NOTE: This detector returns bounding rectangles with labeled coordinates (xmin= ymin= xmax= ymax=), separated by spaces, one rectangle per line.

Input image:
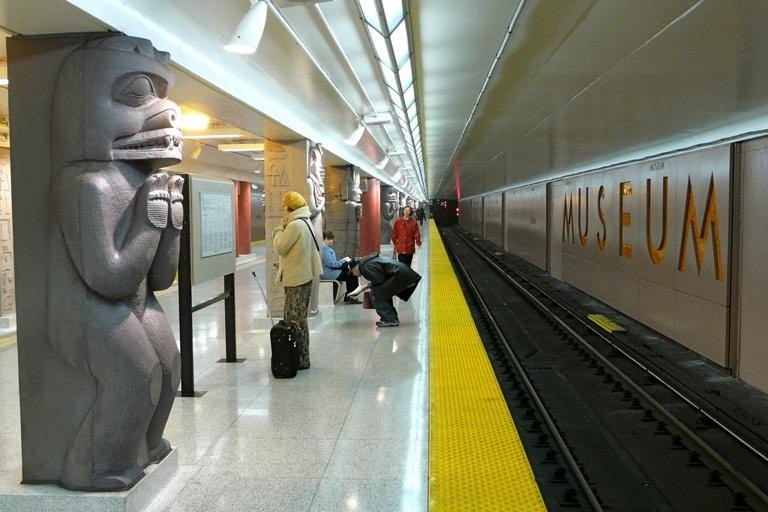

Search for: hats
xmin=282 ymin=192 xmax=306 ymax=210
xmin=349 ymin=258 xmax=360 ymax=269
xmin=324 ymin=231 xmax=335 ymax=239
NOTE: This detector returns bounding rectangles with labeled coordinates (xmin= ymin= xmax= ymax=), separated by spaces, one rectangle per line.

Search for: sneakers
xmin=375 ymin=316 xmax=401 ymax=327
xmin=344 ymin=295 xmax=362 ymax=304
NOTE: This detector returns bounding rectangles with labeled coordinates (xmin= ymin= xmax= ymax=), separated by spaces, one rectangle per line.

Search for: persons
xmin=348 ymin=252 xmax=423 ymax=328
xmin=418 ymin=207 xmax=426 ymax=225
xmin=415 ymin=208 xmax=420 ymax=223
xmin=391 ymin=206 xmax=422 ymax=268
xmin=319 ymin=230 xmax=364 ymax=305
xmin=272 ymin=191 xmax=324 ymax=370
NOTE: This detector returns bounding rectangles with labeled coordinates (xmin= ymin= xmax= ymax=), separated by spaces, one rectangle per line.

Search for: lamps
xmin=224 ymin=1 xmax=268 ymax=55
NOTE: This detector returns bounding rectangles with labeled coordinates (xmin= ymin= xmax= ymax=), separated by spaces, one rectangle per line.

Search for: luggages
xmin=269 ymin=320 xmax=299 ymax=379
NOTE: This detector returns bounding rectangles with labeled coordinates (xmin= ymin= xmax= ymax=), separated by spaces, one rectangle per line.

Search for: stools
xmin=320 ymin=275 xmax=345 ymax=310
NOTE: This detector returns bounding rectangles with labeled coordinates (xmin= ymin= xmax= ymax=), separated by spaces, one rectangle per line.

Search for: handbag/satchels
xmin=363 ymin=291 xmax=375 ymax=310
